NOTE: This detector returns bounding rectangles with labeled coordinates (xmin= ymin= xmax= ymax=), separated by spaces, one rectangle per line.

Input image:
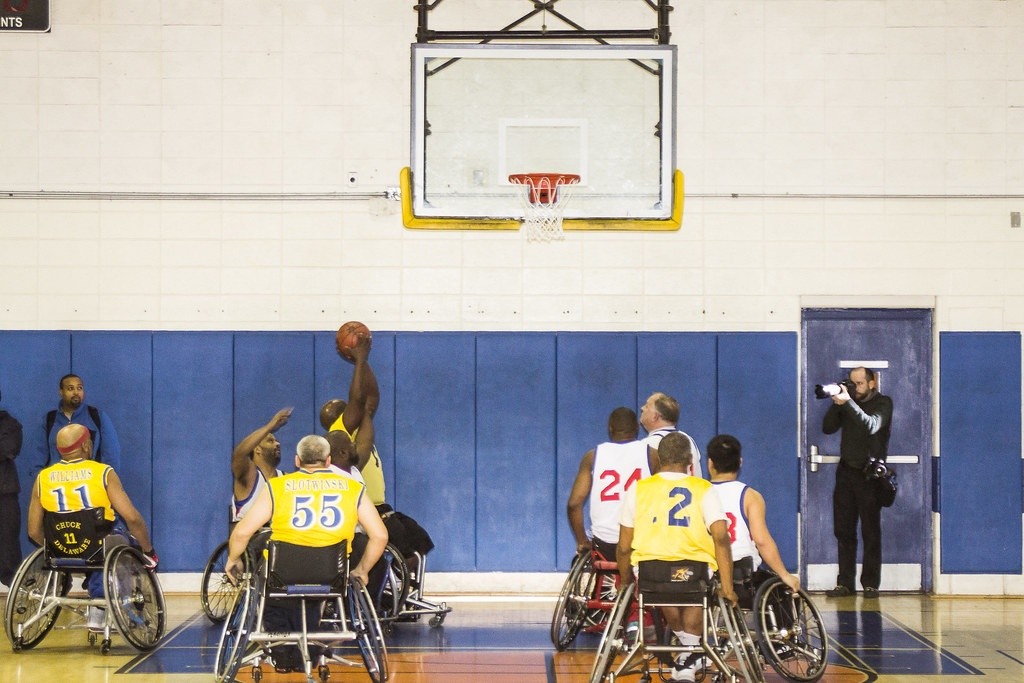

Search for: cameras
xmin=815 ymin=380 xmax=857 ymax=400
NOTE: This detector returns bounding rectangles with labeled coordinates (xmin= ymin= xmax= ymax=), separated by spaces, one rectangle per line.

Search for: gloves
xmin=143 ymin=549 xmax=158 ymax=572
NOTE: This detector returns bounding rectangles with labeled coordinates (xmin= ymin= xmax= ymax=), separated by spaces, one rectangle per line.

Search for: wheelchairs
xmin=202 ymin=505 xmax=453 ymax=639
xmin=588 ymin=559 xmax=766 ymax=683
xmin=550 ymin=538 xmax=826 ymax=683
xmin=214 ymin=538 xmax=390 ymax=683
xmin=4 ymin=506 xmax=167 ymax=655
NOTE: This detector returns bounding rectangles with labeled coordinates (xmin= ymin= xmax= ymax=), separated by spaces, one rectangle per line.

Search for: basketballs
xmin=336 ymin=321 xmax=373 ymax=359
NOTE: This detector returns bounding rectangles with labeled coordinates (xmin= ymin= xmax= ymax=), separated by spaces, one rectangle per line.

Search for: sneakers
xmin=825 ymin=585 xmax=852 ymax=597
xmin=86 ymin=606 xmax=123 ymax=629
xmin=627 ymin=621 xmax=639 ymax=643
xmin=864 ymin=587 xmax=879 ymax=599
xmin=0 ymin=581 xmax=23 ymax=596
xmin=671 ymin=660 xmax=695 ymax=683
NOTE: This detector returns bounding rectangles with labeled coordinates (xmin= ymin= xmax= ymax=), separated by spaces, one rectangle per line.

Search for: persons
xmin=701 ymin=435 xmax=807 ymax=662
xmin=615 ymin=433 xmax=732 ymax=683
xmin=30 ymin=423 xmax=158 ymax=633
xmin=30 ymin=374 xmax=120 ymax=588
xmin=566 ymin=392 xmax=702 ymax=641
xmin=0 ymin=391 xmax=23 ymax=593
xmin=232 ymin=337 xmax=435 ymax=673
xmin=822 ymin=367 xmax=893 ymax=598
